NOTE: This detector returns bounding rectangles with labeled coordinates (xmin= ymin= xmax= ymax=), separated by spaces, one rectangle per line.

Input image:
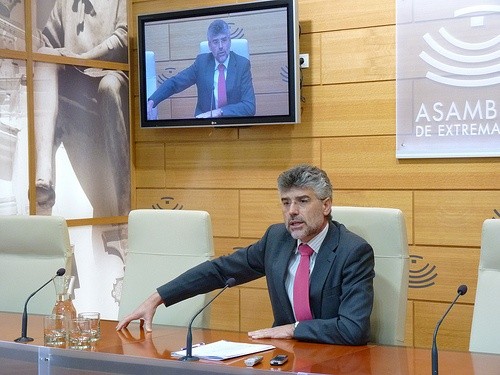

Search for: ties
xmin=217 ymin=63 xmax=227 ymax=108
xmin=293 ymin=243 xmax=315 ymax=321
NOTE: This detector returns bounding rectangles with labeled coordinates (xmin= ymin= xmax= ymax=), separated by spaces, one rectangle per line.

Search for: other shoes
xmin=28 ymin=182 xmax=55 ymax=210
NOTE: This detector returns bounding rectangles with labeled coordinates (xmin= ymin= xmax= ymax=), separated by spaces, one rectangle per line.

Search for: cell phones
xmin=270 ymin=355 xmax=288 ymax=366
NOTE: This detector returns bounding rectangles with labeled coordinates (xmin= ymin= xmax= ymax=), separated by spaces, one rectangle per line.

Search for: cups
xmin=43 ymin=315 xmax=66 ymax=348
xmin=79 ymin=312 xmax=100 ymax=342
xmin=68 ymin=318 xmax=91 ymax=349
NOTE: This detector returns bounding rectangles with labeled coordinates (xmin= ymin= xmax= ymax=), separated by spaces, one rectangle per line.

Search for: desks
xmin=0 ymin=312 xmax=500 ymax=375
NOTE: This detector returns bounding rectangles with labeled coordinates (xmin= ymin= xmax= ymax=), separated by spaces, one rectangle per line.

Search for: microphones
xmin=210 ymin=86 xmax=215 ymax=117
xmin=181 ymin=278 xmax=237 ymax=361
xmin=15 ymin=268 xmax=66 ymax=342
xmin=431 ymin=284 xmax=468 ymax=375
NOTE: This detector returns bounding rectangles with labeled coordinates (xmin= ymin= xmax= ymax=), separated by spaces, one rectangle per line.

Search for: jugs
xmin=51 ymin=274 xmax=76 ymax=338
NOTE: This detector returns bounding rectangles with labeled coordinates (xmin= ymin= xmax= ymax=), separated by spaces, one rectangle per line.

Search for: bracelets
xmin=220 ymin=108 xmax=223 ymax=116
xmin=295 ymin=321 xmax=299 ymax=328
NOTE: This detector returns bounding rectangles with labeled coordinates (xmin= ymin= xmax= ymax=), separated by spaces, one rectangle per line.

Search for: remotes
xmin=244 ymin=356 xmax=263 ymax=366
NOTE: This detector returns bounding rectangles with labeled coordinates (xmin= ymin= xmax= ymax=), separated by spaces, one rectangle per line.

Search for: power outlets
xmin=299 ymin=54 xmax=309 ymax=67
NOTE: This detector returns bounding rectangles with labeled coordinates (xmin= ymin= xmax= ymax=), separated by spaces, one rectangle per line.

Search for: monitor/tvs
xmin=138 ymin=0 xmax=301 ymax=128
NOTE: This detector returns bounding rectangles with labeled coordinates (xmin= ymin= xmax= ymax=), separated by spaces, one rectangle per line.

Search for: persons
xmin=43 ymin=0 xmax=130 ymax=215
xmin=115 ymin=165 xmax=375 ymax=345
xmin=147 ymin=20 xmax=256 ymax=117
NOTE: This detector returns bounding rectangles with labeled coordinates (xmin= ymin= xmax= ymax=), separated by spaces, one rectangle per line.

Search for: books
xmin=171 ymin=340 xmax=276 ymax=361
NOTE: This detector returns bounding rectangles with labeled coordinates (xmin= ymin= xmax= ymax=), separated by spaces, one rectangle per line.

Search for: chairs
xmin=330 ymin=207 xmax=410 ymax=345
xmin=469 ymin=219 xmax=500 ymax=354
xmin=0 ymin=215 xmax=72 ymax=314
xmin=118 ymin=209 xmax=214 ymax=329
